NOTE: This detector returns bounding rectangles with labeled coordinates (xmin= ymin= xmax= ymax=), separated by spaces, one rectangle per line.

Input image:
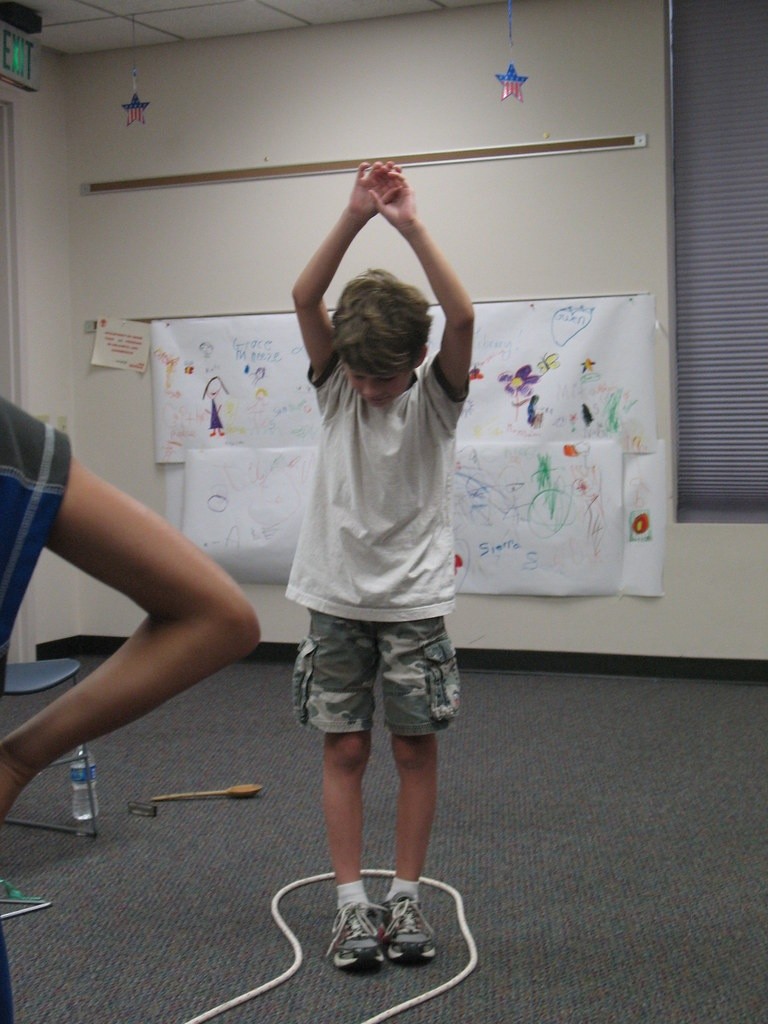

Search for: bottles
xmin=70 ymin=744 xmax=99 ymax=821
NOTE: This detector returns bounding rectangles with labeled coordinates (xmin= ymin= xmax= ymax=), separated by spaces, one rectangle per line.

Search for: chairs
xmin=1 ymin=659 xmax=97 ymax=835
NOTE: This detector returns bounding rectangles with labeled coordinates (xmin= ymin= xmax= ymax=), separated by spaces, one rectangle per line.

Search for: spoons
xmin=151 ymin=784 xmax=263 ymax=802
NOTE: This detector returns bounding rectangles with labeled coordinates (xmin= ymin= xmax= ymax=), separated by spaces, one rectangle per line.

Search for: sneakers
xmin=380 ymin=892 xmax=437 ymax=961
xmin=326 ymin=901 xmax=384 ymax=969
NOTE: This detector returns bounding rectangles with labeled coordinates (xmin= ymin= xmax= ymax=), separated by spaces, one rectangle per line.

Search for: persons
xmin=292 ymin=160 xmax=474 ymax=974
xmin=1 ymin=396 xmax=261 ymax=1024
